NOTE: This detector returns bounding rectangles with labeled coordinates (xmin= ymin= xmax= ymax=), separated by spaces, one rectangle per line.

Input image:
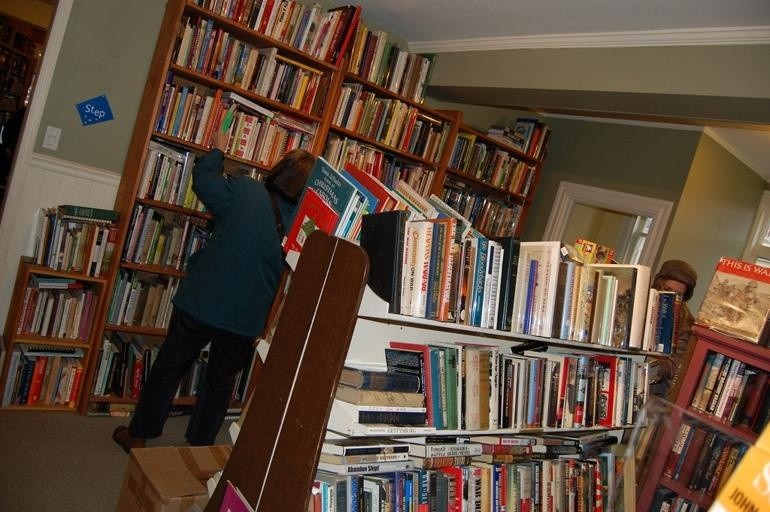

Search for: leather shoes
xmin=113 ymin=425 xmax=145 ymax=453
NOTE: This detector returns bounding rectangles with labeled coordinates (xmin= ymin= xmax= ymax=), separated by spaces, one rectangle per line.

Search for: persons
xmin=112 ymin=129 xmax=315 ymax=459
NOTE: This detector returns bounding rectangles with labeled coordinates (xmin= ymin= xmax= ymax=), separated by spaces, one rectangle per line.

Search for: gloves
xmin=650 ymin=357 xmax=676 ymax=381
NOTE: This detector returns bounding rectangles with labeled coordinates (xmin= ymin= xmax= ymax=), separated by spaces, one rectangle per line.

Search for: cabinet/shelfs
xmin=0 ymin=0 xmax=770 ymax=512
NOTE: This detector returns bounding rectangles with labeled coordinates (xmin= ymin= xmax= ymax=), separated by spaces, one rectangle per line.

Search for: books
xmin=325 ymin=22 xmax=550 ymax=239
xmin=0 ymin=204 xmax=120 ymax=410
xmin=88 ymin=140 xmax=264 ymax=402
xmin=241 ymin=156 xmax=769 ymax=512
xmin=156 ymin=1 xmax=362 ymax=169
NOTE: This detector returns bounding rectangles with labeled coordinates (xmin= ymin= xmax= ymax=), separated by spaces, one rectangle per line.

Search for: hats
xmin=655 ymin=260 xmax=698 ymax=289
